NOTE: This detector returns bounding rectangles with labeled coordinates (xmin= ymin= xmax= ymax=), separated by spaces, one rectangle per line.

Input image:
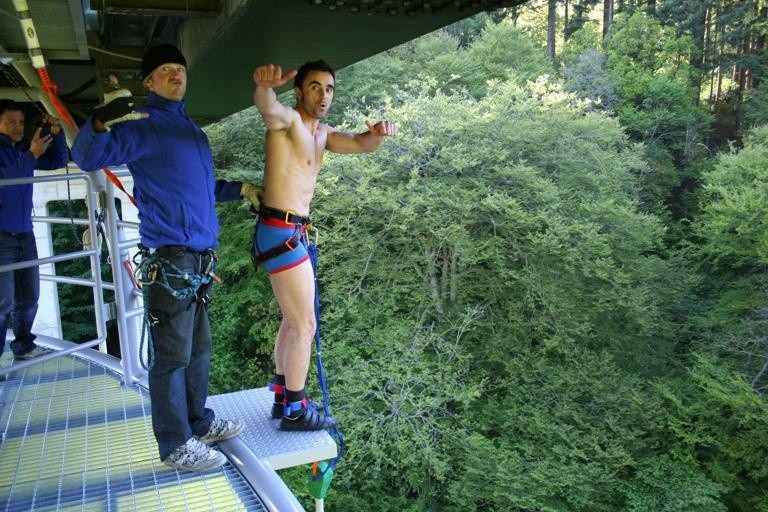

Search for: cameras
xmin=37 ymin=119 xmax=52 ymax=138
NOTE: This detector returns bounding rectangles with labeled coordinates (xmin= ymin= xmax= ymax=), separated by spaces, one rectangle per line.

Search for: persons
xmin=247 ymin=60 xmax=404 ymax=434
xmin=0 ymin=99 xmax=69 ymax=367
xmin=66 ymin=44 xmax=264 ymax=474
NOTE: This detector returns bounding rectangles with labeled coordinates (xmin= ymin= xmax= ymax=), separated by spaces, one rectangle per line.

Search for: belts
xmin=0 ymin=230 xmax=33 ymax=240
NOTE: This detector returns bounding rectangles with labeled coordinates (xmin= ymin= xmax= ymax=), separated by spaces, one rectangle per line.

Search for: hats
xmin=140 ymin=43 xmax=188 ymax=88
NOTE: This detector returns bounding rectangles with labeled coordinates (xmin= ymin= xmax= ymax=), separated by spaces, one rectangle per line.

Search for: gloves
xmin=92 ymin=81 xmax=144 ymax=133
xmin=239 ymin=183 xmax=264 ymax=210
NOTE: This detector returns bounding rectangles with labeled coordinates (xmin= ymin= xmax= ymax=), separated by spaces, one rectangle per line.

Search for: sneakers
xmin=281 ymin=403 xmax=337 ymax=431
xmin=161 ymin=437 xmax=228 ymax=473
xmin=193 ymin=417 xmax=245 ymax=445
xmin=14 ymin=346 xmax=53 ymax=361
xmin=269 ymin=381 xmax=285 ymax=419
